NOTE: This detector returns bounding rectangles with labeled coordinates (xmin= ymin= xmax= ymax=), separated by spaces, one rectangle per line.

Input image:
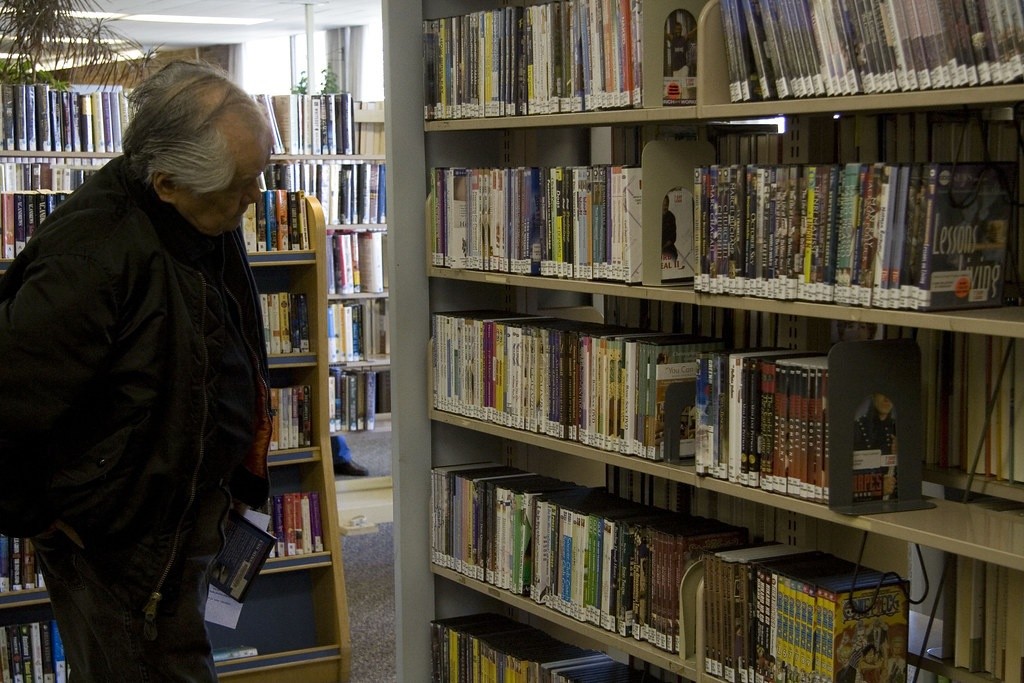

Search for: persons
xmin=667 ymin=23 xmax=697 ymax=78
xmin=0 ymin=59 xmax=274 ymax=683
xmin=853 ymin=392 xmax=897 ymax=500
xmin=662 ymin=195 xmax=678 ymax=262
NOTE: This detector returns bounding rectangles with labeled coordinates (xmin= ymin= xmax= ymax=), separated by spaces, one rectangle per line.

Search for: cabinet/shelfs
xmin=0 ymin=0 xmax=1024 ymax=683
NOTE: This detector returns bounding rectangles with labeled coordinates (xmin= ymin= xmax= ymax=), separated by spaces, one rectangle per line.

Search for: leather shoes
xmin=335 ymin=460 xmax=369 ymax=476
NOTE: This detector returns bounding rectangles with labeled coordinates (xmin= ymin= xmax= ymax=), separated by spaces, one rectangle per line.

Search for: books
xmin=0 ymin=619 xmax=71 ymax=683
xmin=0 ymin=157 xmax=390 ymax=453
xmin=0 ymin=532 xmax=46 ymax=591
xmin=421 ymin=0 xmax=1024 ymax=683
xmin=208 ymin=492 xmax=326 ymax=603
xmin=0 ymin=82 xmax=386 ymax=156
xmin=213 ymin=644 xmax=258 ymax=663
